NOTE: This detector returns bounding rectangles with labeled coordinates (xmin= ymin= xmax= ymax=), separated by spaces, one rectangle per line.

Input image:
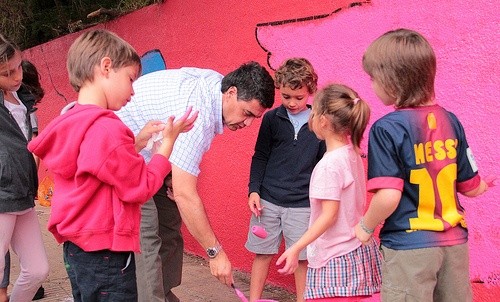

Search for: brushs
xmin=483 ymin=173 xmax=500 ymax=188
xmin=251 ymin=208 xmax=267 ymax=239
xmin=231 ymin=282 xmax=249 ymax=302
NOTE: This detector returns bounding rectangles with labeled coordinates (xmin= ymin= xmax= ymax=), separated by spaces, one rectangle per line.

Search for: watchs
xmin=206 ymin=244 xmax=221 ymax=258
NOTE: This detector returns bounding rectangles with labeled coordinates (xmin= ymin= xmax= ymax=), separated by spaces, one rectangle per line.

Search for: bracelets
xmin=360 ymin=218 xmax=374 ymax=233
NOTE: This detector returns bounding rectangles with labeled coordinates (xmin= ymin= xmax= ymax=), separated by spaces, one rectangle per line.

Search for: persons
xmin=276 ymin=84 xmax=384 ymax=302
xmin=111 ymin=60 xmax=274 ymax=302
xmin=0 ymin=34 xmax=49 ymax=302
xmin=244 ymin=56 xmax=327 ymax=302
xmin=26 ymin=29 xmax=199 ymax=302
xmin=353 ymin=28 xmax=488 ymax=302
